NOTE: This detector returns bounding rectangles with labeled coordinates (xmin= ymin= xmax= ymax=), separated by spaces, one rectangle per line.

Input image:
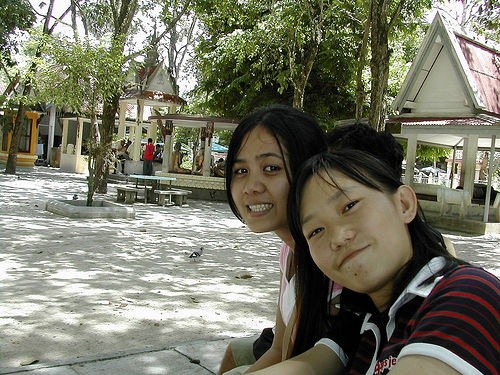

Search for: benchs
xmin=124 ymin=160 xmax=162 ymax=175
xmin=114 ymin=185 xmax=192 ymax=207
xmin=156 ymin=171 xmax=227 ymax=202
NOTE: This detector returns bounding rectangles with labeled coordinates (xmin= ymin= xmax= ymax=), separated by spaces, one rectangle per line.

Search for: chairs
xmin=436 ymin=188 xmax=480 ymax=219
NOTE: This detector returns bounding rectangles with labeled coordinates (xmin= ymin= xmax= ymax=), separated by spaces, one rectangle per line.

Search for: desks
xmin=128 ymin=175 xmax=176 ymax=202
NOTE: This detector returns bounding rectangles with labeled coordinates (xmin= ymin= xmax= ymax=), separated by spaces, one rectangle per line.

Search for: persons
xmin=140 ymin=143 xmax=144 ymax=161
xmin=116 ymin=139 xmax=134 ymax=160
xmin=143 ymin=139 xmax=154 ymax=176
xmin=224 ymin=105 xmax=344 ymax=375
xmin=154 ymin=144 xmax=161 ymax=160
xmin=248 ymin=149 xmax=500 ymax=375
xmin=170 ymin=142 xmax=191 ymax=174
xmin=192 ymin=147 xmax=204 ymax=175
xmin=211 ymin=158 xmax=224 ymax=177
xmin=218 ymin=124 xmax=403 ymax=375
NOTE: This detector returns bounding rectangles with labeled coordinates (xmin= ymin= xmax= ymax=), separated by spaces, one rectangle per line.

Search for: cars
xmin=419 ymin=165 xmax=447 ymax=177
xmin=402 ymin=159 xmax=420 ymax=176
